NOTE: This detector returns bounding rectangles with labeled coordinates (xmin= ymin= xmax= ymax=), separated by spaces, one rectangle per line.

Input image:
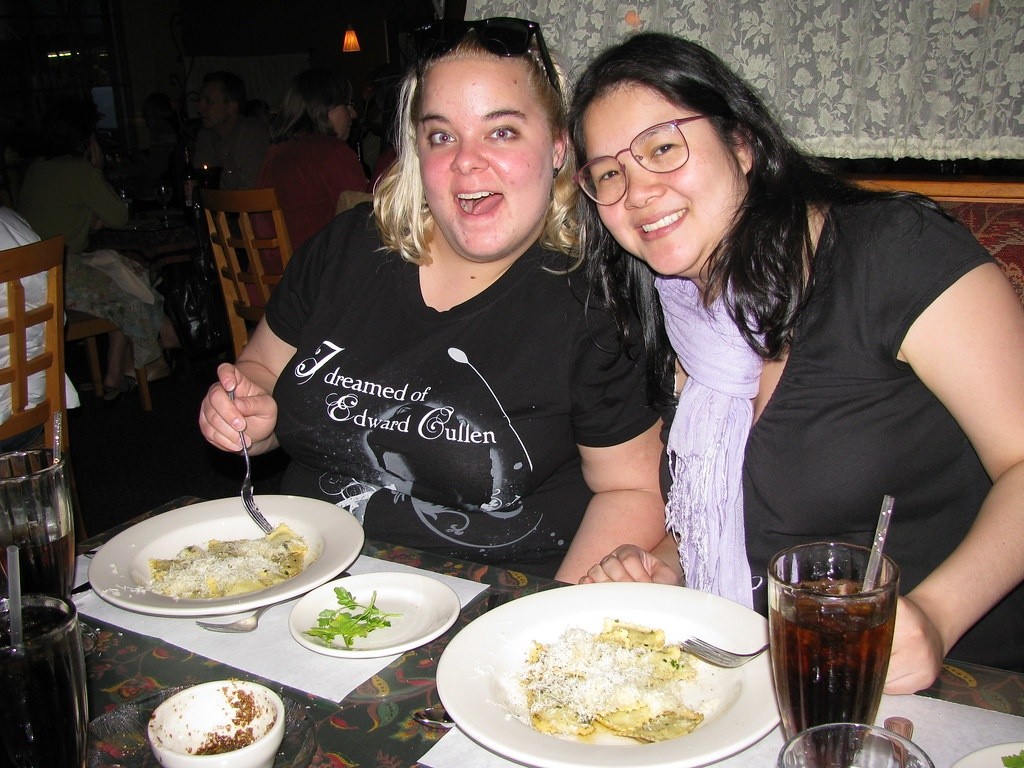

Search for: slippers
xmin=104 ymin=382 xmax=129 ymax=400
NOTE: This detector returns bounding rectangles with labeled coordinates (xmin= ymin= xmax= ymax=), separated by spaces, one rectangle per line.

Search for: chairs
xmin=0 ymin=236 xmax=87 ymax=543
xmin=193 ymin=187 xmax=292 ymax=358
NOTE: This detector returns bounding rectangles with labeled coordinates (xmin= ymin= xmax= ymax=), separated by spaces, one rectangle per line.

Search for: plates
xmin=951 ymin=742 xmax=1024 ymax=768
xmin=88 ymin=497 xmax=365 ymax=615
xmin=288 ymin=573 xmax=461 ymax=659
xmin=436 ymin=583 xmax=782 ymax=768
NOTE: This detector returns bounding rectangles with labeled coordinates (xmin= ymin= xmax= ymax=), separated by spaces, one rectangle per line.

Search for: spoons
xmin=412 ymin=708 xmax=455 ymax=729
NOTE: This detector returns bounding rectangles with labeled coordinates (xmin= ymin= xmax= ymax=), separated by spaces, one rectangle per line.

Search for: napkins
xmin=418 ymin=685 xmax=1024 ymax=768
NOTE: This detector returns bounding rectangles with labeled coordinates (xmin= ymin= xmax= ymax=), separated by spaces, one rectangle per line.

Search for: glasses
xmin=572 ymin=112 xmax=726 ymax=207
xmin=409 ymin=17 xmax=562 ymax=96
xmin=337 ymin=101 xmax=355 ymax=113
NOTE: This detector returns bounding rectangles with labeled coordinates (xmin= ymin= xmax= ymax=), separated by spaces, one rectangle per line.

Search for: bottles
xmin=179 ymin=145 xmax=200 ymax=206
xmin=355 ymin=141 xmax=369 ymax=178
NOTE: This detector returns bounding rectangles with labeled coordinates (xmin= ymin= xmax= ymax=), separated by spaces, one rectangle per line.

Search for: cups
xmin=117 ymin=188 xmax=134 ymax=208
xmin=0 ymin=448 xmax=76 ymax=604
xmin=767 ymin=541 xmax=900 ymax=768
xmin=0 ymin=593 xmax=90 ymax=767
xmin=777 ymin=722 xmax=935 ymax=768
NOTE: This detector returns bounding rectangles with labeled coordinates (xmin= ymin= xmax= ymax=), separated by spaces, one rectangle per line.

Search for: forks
xmin=229 ymin=391 xmax=274 ymax=535
xmin=195 ymin=572 xmax=351 ymax=634
xmin=681 ymin=636 xmax=769 ymax=668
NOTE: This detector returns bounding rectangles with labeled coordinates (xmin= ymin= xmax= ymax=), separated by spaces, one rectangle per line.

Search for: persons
xmin=0 ymin=67 xmax=370 ymax=453
xmin=566 ymin=33 xmax=1024 ymax=695
xmin=199 ymin=17 xmax=666 ymax=585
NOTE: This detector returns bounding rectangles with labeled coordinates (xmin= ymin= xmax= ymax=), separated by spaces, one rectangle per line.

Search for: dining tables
xmin=65 ymin=492 xmax=1024 ymax=768
xmin=69 ymin=543 xmax=491 ymax=705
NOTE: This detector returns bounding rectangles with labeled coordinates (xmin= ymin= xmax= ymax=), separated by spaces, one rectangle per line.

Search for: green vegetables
xmin=304 ymin=586 xmax=404 ymax=649
xmin=1001 ymin=750 xmax=1024 ymax=768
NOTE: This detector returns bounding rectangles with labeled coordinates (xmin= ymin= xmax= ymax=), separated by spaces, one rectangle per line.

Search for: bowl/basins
xmin=148 ymin=679 xmax=285 ymax=767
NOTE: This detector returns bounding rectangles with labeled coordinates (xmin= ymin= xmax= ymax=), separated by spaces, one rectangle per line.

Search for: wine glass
xmin=153 ymin=180 xmax=173 ymax=225
